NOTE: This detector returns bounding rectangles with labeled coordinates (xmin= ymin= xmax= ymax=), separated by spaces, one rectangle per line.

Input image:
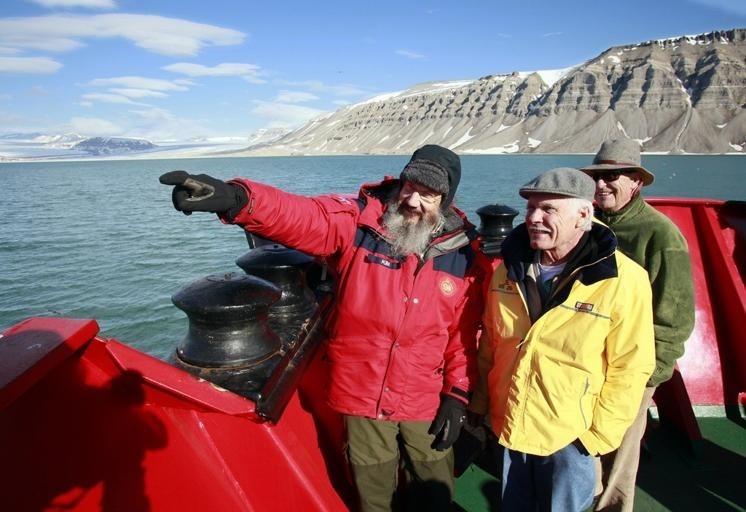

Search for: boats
xmin=0 ymin=197 xmax=746 ymax=510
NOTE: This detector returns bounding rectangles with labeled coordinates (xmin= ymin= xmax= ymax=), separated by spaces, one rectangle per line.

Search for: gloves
xmin=158 ymin=170 xmax=239 ymax=216
xmin=428 ymin=395 xmax=467 ymax=451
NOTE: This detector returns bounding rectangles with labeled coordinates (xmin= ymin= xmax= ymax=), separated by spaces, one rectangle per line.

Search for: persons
xmin=575 ymin=136 xmax=695 ymax=512
xmin=158 ymin=142 xmax=494 ymax=512
xmin=476 ymin=165 xmax=658 ymax=512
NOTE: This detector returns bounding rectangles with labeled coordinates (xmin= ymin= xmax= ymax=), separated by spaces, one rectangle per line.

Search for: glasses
xmin=590 ymin=168 xmax=638 ymax=182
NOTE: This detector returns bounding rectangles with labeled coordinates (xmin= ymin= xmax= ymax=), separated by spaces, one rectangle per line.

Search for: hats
xmin=519 ymin=167 xmax=597 ymax=200
xmin=399 ymin=144 xmax=462 ymax=212
xmin=579 ymin=139 xmax=655 ymax=187
